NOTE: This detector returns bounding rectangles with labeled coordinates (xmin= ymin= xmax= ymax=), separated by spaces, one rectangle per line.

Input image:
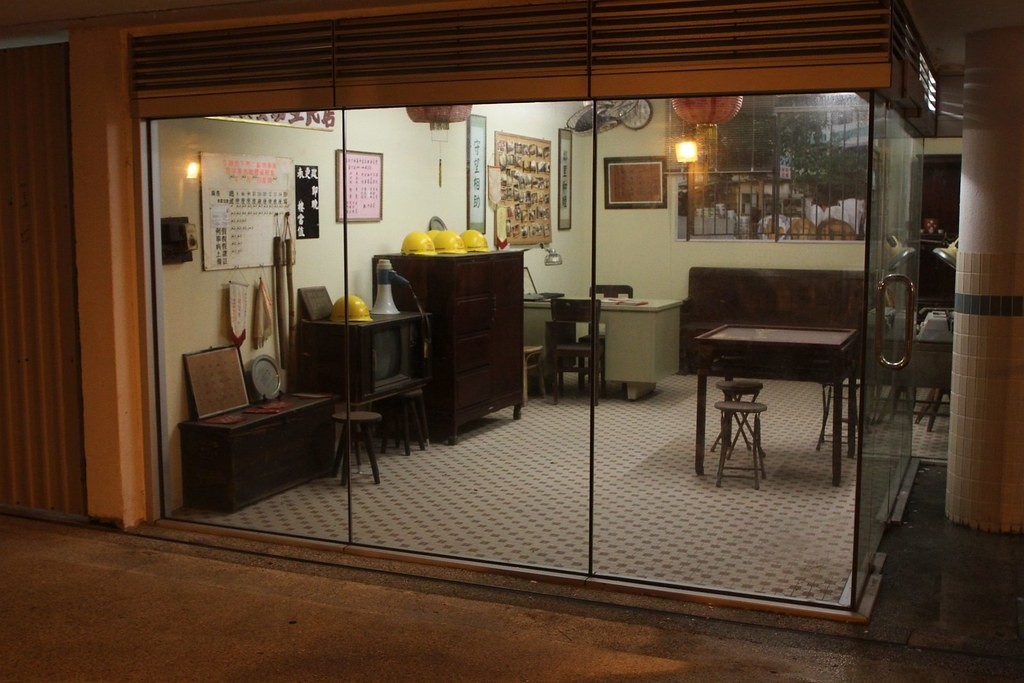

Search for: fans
xmin=566 ymin=99 xmax=640 ymax=136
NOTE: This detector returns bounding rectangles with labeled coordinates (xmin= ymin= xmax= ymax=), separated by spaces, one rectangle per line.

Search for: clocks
xmin=619 ymin=99 xmax=653 ymax=130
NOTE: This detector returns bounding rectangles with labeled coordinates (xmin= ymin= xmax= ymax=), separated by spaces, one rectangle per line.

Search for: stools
xmin=711 ymin=381 xmax=766 ymax=457
xmin=381 ymin=389 xmax=425 ymax=456
xmin=816 ymin=378 xmax=864 ymax=458
xmin=331 ymin=411 xmax=382 ymax=485
xmin=714 ymin=401 xmax=767 ymax=490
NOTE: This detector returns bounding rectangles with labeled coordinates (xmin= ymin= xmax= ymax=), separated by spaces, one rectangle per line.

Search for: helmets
xmin=401 ymin=229 xmax=490 ymax=255
xmin=329 ymin=295 xmax=373 ymax=323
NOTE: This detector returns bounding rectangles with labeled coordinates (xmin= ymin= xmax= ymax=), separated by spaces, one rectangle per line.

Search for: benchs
xmin=680 ymin=266 xmax=865 ymax=379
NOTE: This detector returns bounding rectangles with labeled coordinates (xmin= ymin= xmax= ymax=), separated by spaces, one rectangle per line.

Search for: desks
xmin=523 ymin=296 xmax=682 ymax=400
xmin=694 ymin=324 xmax=859 ymax=485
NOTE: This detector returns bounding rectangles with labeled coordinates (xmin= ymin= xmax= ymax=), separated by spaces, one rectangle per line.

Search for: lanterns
xmin=670 ymin=96 xmax=743 ymax=169
xmin=405 ymin=105 xmax=471 ymax=189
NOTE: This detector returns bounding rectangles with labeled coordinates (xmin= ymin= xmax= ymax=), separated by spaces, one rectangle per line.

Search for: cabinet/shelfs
xmin=371 ymin=250 xmax=523 ymax=445
xmin=177 ymin=392 xmax=336 ymax=512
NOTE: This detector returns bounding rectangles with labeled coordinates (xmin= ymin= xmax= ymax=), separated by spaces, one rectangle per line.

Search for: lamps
xmin=524 ymin=242 xmax=562 ymax=266
xmin=932 ymin=238 xmax=958 ymax=269
xmin=881 ymin=236 xmax=916 ymax=307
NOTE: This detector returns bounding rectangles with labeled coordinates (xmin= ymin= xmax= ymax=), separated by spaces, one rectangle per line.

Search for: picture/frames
xmin=604 ymin=155 xmax=668 ymax=209
xmin=183 ymin=345 xmax=249 ymax=421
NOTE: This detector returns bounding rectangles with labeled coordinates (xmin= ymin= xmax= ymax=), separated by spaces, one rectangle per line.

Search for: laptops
xmin=524 ymin=267 xmax=565 ymax=299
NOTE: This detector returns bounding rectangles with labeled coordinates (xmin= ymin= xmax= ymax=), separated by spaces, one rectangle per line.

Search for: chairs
xmin=578 ymin=285 xmax=634 ymax=393
xmin=550 ymin=298 xmax=605 ymax=407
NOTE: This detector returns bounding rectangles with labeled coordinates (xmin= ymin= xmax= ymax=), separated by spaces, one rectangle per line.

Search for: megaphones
xmin=368 ymin=260 xmax=410 ymax=314
